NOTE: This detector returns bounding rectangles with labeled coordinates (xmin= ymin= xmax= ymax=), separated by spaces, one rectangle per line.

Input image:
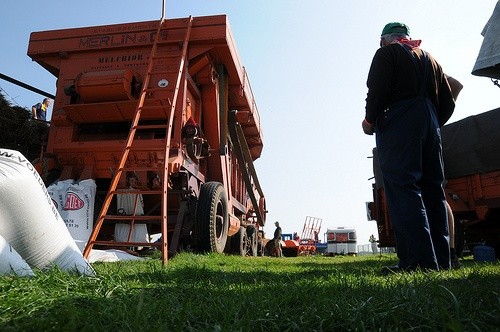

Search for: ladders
xmin=82 ymin=15 xmax=193 ymax=269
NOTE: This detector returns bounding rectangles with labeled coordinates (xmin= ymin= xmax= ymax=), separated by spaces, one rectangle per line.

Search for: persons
xmin=369 ymin=234 xmax=377 ymax=254
xmin=31 ymin=97 xmax=51 ymax=121
xmin=274 ymin=221 xmax=283 ymax=257
xmin=361 ymin=22 xmax=456 ymax=273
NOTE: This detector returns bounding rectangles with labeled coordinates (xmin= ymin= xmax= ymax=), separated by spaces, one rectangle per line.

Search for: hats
xmin=380 ymin=22 xmax=410 ymax=46
